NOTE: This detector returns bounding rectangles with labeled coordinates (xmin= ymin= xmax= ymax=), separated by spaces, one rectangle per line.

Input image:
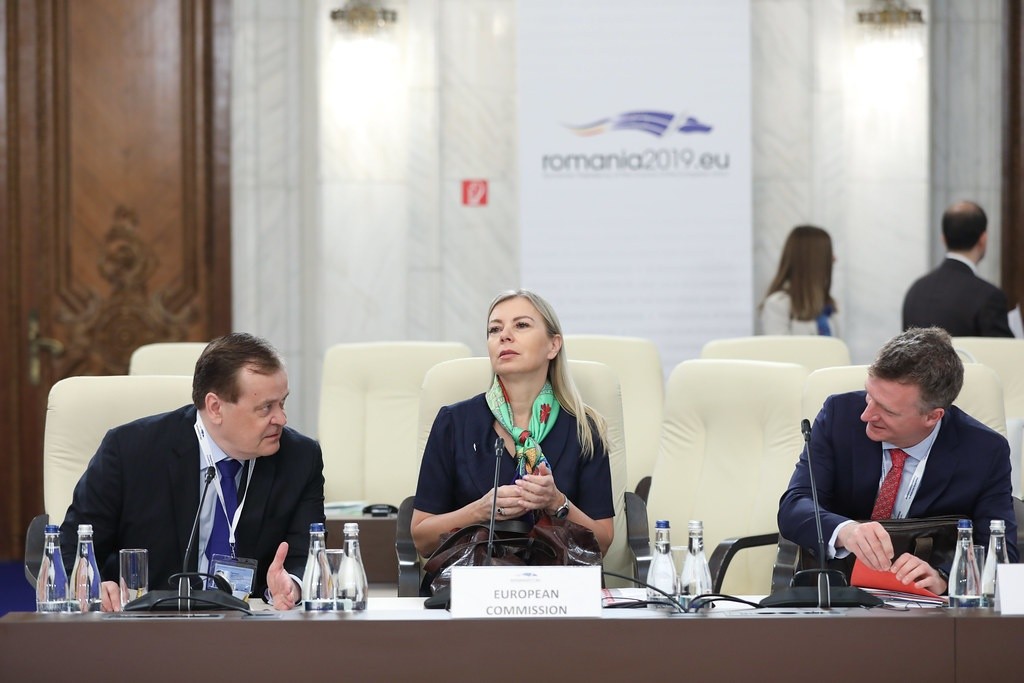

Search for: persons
xmin=776 ymin=325 xmax=1019 ymax=596
xmin=901 ymin=199 xmax=1016 ymax=337
xmin=760 ymin=225 xmax=838 ymax=338
xmin=409 ymin=288 xmax=616 ymax=597
xmin=48 ymin=331 xmax=327 ymax=612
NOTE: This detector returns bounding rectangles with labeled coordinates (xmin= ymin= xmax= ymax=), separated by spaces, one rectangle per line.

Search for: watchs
xmin=545 ymin=493 xmax=570 ymax=521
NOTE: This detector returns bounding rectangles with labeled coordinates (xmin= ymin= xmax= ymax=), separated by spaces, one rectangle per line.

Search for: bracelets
xmin=937 ymin=567 xmax=949 ymax=586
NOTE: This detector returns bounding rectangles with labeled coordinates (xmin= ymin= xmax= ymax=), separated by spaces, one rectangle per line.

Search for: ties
xmin=870 ymin=448 xmax=910 ymax=520
xmin=204 ymin=459 xmax=242 ymax=590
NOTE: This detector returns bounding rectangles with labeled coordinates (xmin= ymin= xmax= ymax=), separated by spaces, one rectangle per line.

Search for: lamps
xmin=330 ymin=1 xmax=396 ymax=39
xmin=857 ymin=0 xmax=925 ymax=34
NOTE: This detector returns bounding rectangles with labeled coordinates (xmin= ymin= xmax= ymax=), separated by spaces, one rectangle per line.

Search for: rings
xmin=496 ymin=507 xmax=506 ymax=516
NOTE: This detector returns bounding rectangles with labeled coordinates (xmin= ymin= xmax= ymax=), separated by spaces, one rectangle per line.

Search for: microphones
xmin=121 ymin=465 xmax=251 ymax=611
xmin=424 ymin=437 xmax=506 ymax=611
xmin=760 ymin=419 xmax=884 ymax=607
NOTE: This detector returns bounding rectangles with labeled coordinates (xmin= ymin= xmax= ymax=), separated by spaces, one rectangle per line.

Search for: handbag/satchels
xmin=801 ymin=515 xmax=974 ymax=586
xmin=424 ymin=517 xmax=605 ymax=609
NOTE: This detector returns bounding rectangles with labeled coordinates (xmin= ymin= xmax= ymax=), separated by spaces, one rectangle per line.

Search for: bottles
xmin=645 ymin=521 xmax=678 ymax=610
xmin=302 ymin=522 xmax=333 ymax=610
xmin=333 ymin=523 xmax=368 ymax=610
xmin=679 ymin=520 xmax=713 ymax=611
xmin=979 ymin=519 xmax=1009 ymax=607
xmin=35 ymin=525 xmax=68 ymax=613
xmin=948 ymin=519 xmax=980 ymax=607
xmin=69 ymin=525 xmax=102 ymax=614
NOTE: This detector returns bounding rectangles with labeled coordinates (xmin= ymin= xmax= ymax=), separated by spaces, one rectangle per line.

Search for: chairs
xmin=315 ymin=333 xmax=1024 ymax=598
xmin=42 ymin=336 xmax=209 ymax=529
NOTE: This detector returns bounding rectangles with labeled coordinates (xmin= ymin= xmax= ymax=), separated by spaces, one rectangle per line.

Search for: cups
xmin=974 ymin=545 xmax=984 ymax=572
xmin=324 ymin=547 xmax=344 ymax=583
xmin=120 ymin=548 xmax=149 ymax=611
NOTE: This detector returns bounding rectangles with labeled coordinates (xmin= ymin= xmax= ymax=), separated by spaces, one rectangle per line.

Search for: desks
xmin=1 ymin=596 xmax=1023 ymax=683
